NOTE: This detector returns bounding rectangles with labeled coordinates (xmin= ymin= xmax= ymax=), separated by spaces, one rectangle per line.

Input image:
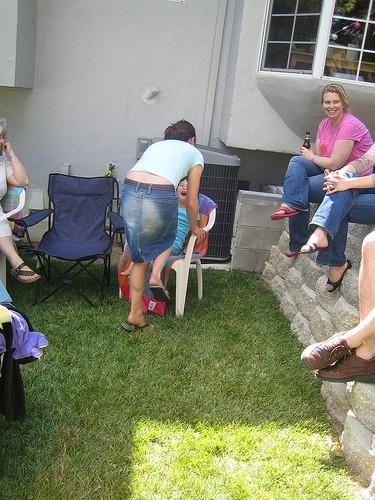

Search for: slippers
xmin=287 ymin=249 xmax=300 ymax=257
xmin=327 ymin=260 xmax=351 ymax=293
xmin=149 ymin=286 xmax=170 ymax=301
xmin=120 ymin=322 xmax=154 ymax=331
xmin=11 ymin=263 xmax=41 ymax=283
xmin=271 ymin=207 xmax=299 ymax=220
xmin=299 ymin=242 xmax=329 ymax=254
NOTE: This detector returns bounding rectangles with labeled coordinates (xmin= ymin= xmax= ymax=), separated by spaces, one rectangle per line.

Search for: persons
xmin=0 ymin=118 xmax=41 ymax=283
xmin=300 ymin=143 xmax=375 ymax=292
xmin=301 ymin=231 xmax=375 ymax=382
xmin=271 ymin=84 xmax=374 ymax=257
xmin=121 ymin=177 xmax=201 ymax=279
xmin=120 ymin=119 xmax=207 ymax=332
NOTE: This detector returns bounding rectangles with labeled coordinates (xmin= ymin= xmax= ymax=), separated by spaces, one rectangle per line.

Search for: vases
xmin=346 ymin=43 xmax=360 ymax=59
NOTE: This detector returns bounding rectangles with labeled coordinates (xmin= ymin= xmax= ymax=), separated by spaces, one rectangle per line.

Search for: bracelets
xmin=11 ymin=154 xmax=15 ymax=158
xmin=312 ymin=157 xmax=316 ymax=161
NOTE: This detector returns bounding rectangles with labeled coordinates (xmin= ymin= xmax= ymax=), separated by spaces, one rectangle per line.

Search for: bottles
xmin=303 ymin=132 xmax=311 ymax=151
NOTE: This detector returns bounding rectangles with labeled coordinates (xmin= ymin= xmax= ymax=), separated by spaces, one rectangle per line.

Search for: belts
xmin=124 ymin=178 xmax=175 ymax=191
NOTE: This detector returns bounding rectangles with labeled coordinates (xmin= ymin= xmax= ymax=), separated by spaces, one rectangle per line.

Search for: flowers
xmin=344 ymin=21 xmax=363 ymax=44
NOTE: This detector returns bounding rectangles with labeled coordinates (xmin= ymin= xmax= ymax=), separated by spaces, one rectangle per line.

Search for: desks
xmin=289 ymin=51 xmax=375 ymax=82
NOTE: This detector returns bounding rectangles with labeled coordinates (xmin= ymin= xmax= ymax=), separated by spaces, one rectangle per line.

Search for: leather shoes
xmin=300 ymin=331 xmax=356 ymax=370
xmin=315 ymin=351 xmax=375 ymax=383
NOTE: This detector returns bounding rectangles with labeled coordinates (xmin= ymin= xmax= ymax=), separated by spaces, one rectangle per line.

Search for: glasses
xmin=0 ymin=134 xmax=7 ymax=141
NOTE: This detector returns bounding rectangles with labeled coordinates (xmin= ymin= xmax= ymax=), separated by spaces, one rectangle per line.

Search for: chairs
xmin=0 ymin=183 xmax=26 ymax=238
xmin=8 ymin=172 xmax=125 ymax=307
xmin=119 ymin=192 xmax=217 ymax=317
